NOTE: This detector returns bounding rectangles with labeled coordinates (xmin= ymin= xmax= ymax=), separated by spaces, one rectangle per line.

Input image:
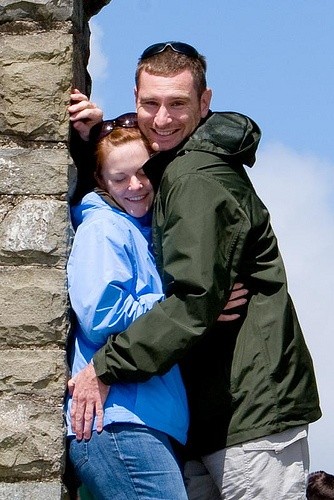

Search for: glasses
xmin=89 ymin=112 xmax=139 ymax=156
xmin=141 ymin=43 xmax=205 ymax=71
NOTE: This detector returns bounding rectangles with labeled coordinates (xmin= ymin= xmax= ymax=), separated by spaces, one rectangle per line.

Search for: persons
xmin=66 ymin=112 xmax=248 ymax=500
xmin=68 ymin=42 xmax=322 ymax=500
